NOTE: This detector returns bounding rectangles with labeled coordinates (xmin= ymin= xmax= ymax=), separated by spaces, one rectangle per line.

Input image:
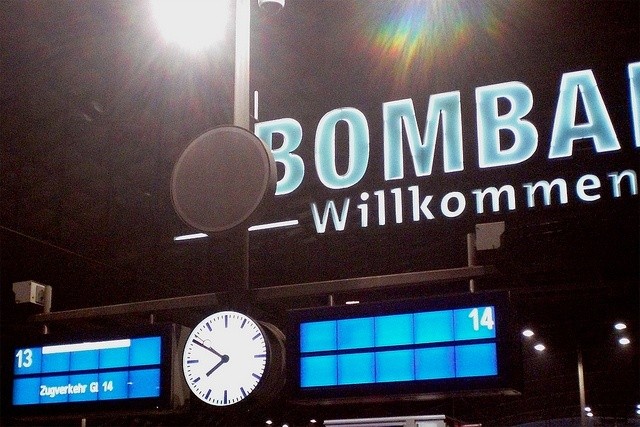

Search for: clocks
xmin=181 ymin=310 xmax=267 ymax=407
